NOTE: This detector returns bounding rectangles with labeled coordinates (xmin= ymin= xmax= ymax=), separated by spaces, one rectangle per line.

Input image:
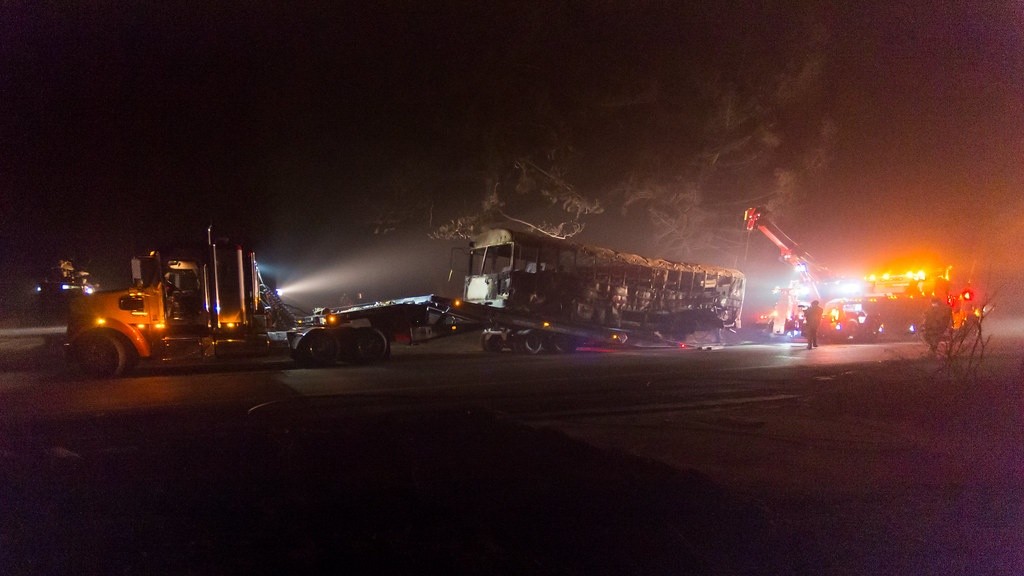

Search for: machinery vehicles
xmin=735 ymin=206 xmax=838 ymax=329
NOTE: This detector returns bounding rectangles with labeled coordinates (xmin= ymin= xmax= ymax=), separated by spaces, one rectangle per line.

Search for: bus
xmin=448 ymin=217 xmax=745 ymax=334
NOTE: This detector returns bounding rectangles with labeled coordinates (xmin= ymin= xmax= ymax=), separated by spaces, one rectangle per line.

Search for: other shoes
xmin=813 ymin=345 xmax=817 ymax=347
xmin=807 ymin=346 xmax=812 ymax=349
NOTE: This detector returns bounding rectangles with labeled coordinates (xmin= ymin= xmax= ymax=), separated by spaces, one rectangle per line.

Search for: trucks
xmin=61 ymin=221 xmax=625 ymax=380
xmin=818 ymin=266 xmax=981 ymax=344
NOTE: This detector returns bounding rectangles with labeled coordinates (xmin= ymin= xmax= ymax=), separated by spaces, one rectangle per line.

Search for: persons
xmin=802 ymin=300 xmax=824 ymax=350
